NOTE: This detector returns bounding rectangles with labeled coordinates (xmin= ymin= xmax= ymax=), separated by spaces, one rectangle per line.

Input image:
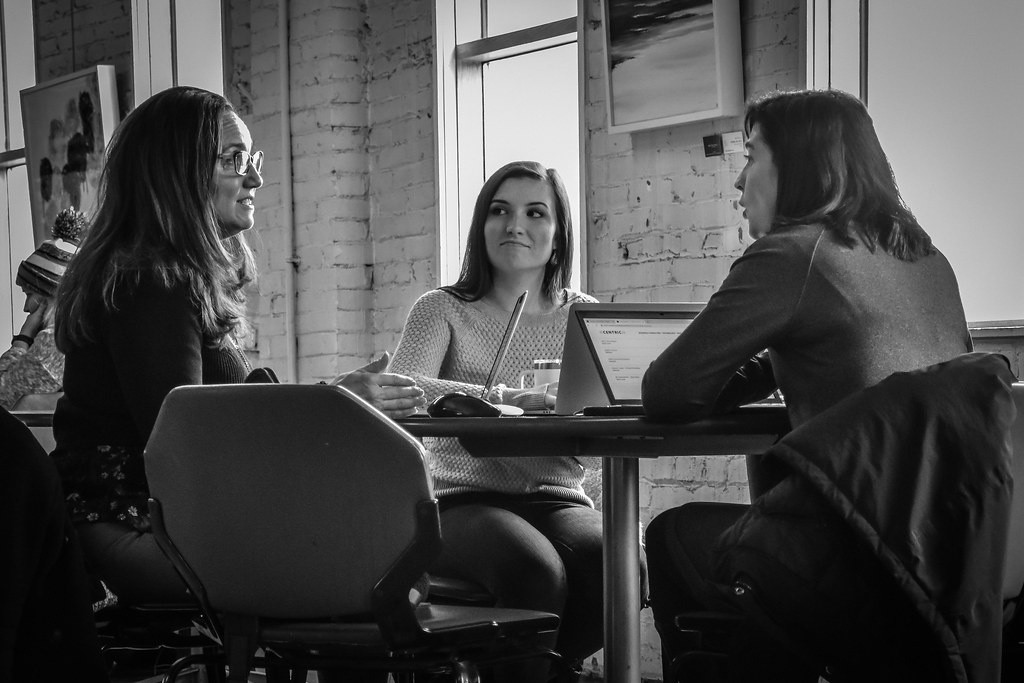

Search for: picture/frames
xmin=600 ymin=0 xmax=745 ymax=137
xmin=20 ymin=64 xmax=121 ymax=250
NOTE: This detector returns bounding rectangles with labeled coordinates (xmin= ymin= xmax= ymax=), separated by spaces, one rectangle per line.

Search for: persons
xmin=384 ymin=160 xmax=649 ymax=683
xmin=641 ymin=87 xmax=973 ymax=683
xmin=46 ymin=86 xmax=424 ymax=604
xmin=0 ymin=206 xmax=92 ymax=410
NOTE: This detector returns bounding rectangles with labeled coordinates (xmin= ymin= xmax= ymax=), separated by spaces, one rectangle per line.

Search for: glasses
xmin=217 ymin=151 xmax=264 ymax=176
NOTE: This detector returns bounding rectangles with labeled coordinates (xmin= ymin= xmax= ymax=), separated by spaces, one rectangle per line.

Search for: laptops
xmin=573 ymin=302 xmax=788 ymax=412
xmin=408 ymin=292 xmax=527 ymax=417
xmin=520 ymin=306 xmax=611 ymax=416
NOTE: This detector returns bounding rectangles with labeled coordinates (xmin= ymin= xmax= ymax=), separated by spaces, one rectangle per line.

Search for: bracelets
xmin=11 ymin=333 xmax=33 ymax=347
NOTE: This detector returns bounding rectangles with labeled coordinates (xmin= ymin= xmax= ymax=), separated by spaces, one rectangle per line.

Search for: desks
xmin=397 ymin=409 xmax=791 ymax=683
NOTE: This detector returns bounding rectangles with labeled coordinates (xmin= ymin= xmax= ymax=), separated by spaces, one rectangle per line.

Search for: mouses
xmin=427 ymin=393 xmax=502 ymax=417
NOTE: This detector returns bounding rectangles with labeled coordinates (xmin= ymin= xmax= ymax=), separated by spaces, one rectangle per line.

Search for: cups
xmin=518 ymin=359 xmax=563 ymax=390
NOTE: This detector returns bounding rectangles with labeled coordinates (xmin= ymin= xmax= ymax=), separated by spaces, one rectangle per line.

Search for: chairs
xmin=667 ymin=351 xmax=1024 ymax=683
xmin=0 ymin=405 xmax=227 ymax=683
xmin=144 ymin=382 xmax=562 ymax=683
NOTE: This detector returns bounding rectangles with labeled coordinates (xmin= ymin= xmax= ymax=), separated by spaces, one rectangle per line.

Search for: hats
xmin=16 ymin=206 xmax=90 ymax=299
